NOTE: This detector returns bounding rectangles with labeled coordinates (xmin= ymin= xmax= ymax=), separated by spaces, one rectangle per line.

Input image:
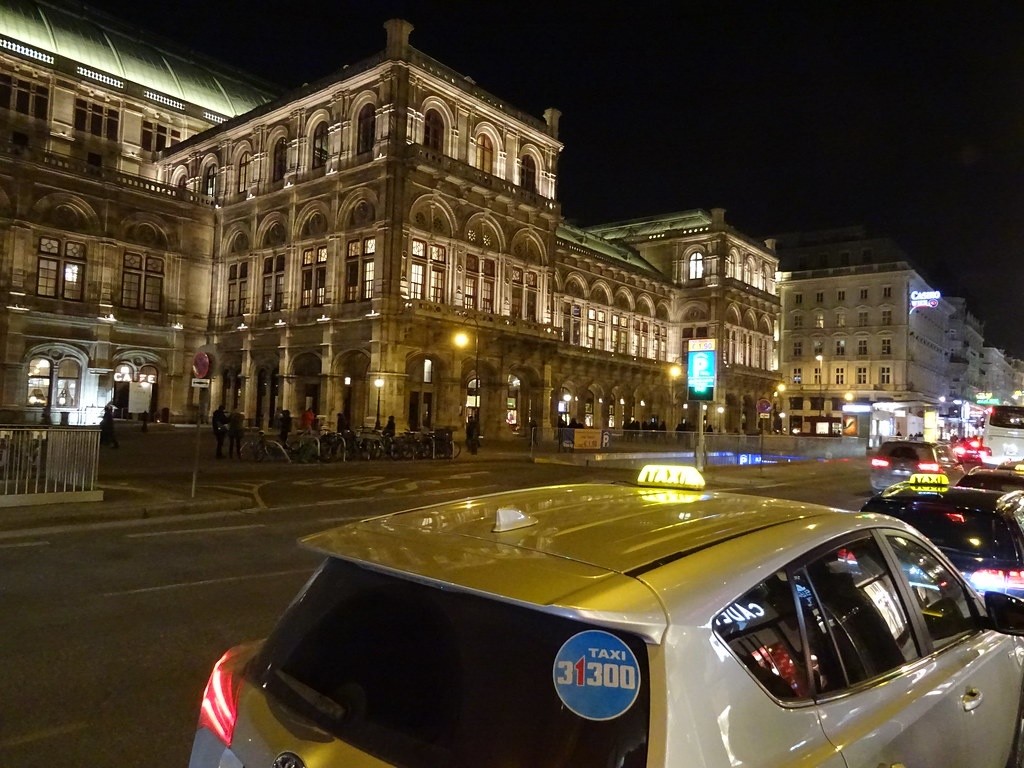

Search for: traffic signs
xmin=686 ymin=339 xmax=719 ymax=379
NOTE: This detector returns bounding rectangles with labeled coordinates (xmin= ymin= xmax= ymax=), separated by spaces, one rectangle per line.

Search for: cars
xmin=955 ymin=439 xmax=982 ymax=463
xmin=954 ymin=466 xmax=1024 ymax=492
xmin=857 ymin=474 xmax=1024 ymax=596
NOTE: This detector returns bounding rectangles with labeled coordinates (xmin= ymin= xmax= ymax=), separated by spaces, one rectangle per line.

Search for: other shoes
xmin=112 ymin=445 xmax=120 ymax=449
xmin=229 ymin=452 xmax=233 ymax=458
xmin=216 ymin=454 xmax=224 ymax=458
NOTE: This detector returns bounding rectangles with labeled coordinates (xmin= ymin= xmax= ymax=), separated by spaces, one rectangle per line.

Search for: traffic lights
xmin=688 ymin=386 xmax=714 ymax=401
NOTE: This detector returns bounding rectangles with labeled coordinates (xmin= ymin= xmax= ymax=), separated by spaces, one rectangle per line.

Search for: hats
xmin=233 ymin=409 xmax=239 ymax=414
xmin=219 ymin=406 xmax=225 ymax=410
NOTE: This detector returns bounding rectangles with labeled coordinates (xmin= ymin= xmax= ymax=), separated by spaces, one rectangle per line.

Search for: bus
xmin=980 ymin=404 xmax=1024 ymax=468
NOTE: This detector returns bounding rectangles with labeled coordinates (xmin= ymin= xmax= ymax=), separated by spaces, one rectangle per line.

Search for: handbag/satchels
xmin=216 ymin=425 xmax=226 ymax=433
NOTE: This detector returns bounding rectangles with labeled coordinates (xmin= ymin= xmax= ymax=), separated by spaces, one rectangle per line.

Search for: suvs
xmin=870 ymin=436 xmax=967 ymax=496
xmin=186 ymin=464 xmax=1023 ymax=767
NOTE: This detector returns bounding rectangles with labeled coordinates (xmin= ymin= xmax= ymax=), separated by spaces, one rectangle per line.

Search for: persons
xmin=100 ymin=407 xmax=121 ymax=450
xmin=334 ymin=413 xmax=345 ymax=453
xmin=228 ymin=409 xmax=245 ymax=459
xmin=304 ymin=407 xmax=316 ymax=434
xmin=528 ymin=420 xmax=540 ymax=448
xmin=909 ymin=432 xmax=923 ymax=441
xmin=212 ymin=405 xmax=231 ymax=459
xmin=465 ymin=416 xmax=482 ymax=455
xmin=675 ymin=422 xmax=696 ymax=443
xmin=622 ymin=418 xmax=631 ymax=440
xmin=578 ymin=423 xmax=584 ymax=429
xmin=642 ymin=418 xmax=666 ymax=444
xmin=382 ymin=415 xmax=395 ymax=437
xmin=705 ymin=424 xmax=713 ymax=432
xmin=278 ymin=410 xmax=293 ymax=444
xmin=630 ymin=421 xmax=640 ymax=441
xmin=772 ymin=411 xmax=782 ymax=435
xmin=558 ymin=415 xmax=567 ymax=453
xmin=567 ymin=418 xmax=578 ymax=453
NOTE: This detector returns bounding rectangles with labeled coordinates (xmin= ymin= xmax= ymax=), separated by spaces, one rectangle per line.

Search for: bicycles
xmin=239 ymin=429 xmax=462 ymax=463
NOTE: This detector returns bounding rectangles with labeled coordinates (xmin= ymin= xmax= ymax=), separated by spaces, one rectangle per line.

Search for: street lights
xmin=816 ymin=355 xmax=822 ymax=420
xmin=375 ymin=375 xmax=383 ymax=428
xmin=454 ymin=317 xmax=479 ymax=454
